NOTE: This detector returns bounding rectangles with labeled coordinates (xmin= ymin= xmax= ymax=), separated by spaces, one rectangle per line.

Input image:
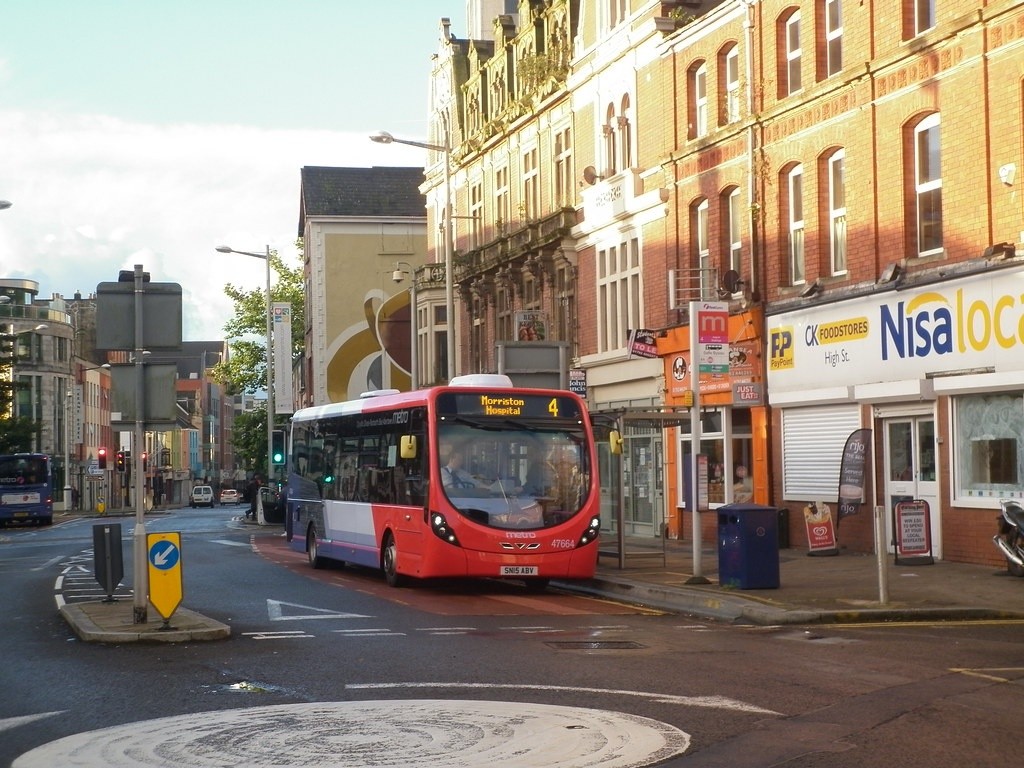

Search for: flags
xmin=839 ymin=429 xmax=871 ymax=518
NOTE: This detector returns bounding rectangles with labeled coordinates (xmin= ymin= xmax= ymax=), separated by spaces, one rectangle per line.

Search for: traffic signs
xmin=86 ymin=465 xmax=105 ymax=477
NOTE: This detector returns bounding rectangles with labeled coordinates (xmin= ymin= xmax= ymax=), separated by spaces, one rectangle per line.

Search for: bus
xmin=285 ymin=374 xmax=624 ymax=594
xmin=0 ymin=453 xmax=54 ymax=528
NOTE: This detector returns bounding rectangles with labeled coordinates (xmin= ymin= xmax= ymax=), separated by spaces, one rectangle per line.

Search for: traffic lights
xmin=142 ymin=452 xmax=148 ymax=473
xmin=271 ymin=429 xmax=286 ymax=465
xmin=117 ymin=451 xmax=127 ymax=474
xmin=98 ymin=448 xmax=107 ymax=469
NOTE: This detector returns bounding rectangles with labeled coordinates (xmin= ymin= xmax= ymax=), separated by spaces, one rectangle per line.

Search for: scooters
xmin=992 ymin=499 xmax=1024 ymax=578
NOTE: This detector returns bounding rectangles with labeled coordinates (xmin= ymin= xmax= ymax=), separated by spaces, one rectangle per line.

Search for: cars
xmin=257 ymin=486 xmax=285 ymax=526
xmin=220 ymin=489 xmax=240 ymax=506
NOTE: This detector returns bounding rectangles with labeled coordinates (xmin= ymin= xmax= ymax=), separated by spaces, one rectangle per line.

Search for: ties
xmin=452 ymin=471 xmax=458 ymax=488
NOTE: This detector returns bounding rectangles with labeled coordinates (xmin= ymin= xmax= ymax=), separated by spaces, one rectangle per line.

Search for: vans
xmin=192 ymin=486 xmax=214 ymax=508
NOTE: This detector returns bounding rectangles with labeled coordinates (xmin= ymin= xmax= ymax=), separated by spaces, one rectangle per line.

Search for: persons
xmin=246 ymin=473 xmax=260 ymax=520
xmin=440 ymin=449 xmax=489 ymax=489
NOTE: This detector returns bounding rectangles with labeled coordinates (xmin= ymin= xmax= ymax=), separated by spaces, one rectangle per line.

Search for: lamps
xmin=982 ymin=242 xmax=1018 ymax=264
xmin=798 ymin=282 xmax=822 ymax=297
xmin=880 ymin=263 xmax=904 ymax=284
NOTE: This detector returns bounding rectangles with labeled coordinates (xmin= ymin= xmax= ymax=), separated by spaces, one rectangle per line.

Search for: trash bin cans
xmin=716 ymin=503 xmax=780 ymax=589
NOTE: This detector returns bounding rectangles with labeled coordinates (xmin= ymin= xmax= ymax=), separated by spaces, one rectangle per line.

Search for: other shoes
xmin=245 ymin=510 xmax=250 ymax=519
xmin=252 ymin=516 xmax=257 ymax=520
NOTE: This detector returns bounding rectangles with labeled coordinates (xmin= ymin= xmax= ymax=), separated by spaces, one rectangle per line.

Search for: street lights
xmin=63 ymin=385 xmax=73 ymax=513
xmin=79 ymin=364 xmax=112 ymax=511
xmin=392 ymin=261 xmax=418 ymax=392
xmin=370 ymin=129 xmax=457 ymax=386
xmin=8 ymin=324 xmax=49 ymax=417
xmin=215 ymin=245 xmax=275 ymax=494
